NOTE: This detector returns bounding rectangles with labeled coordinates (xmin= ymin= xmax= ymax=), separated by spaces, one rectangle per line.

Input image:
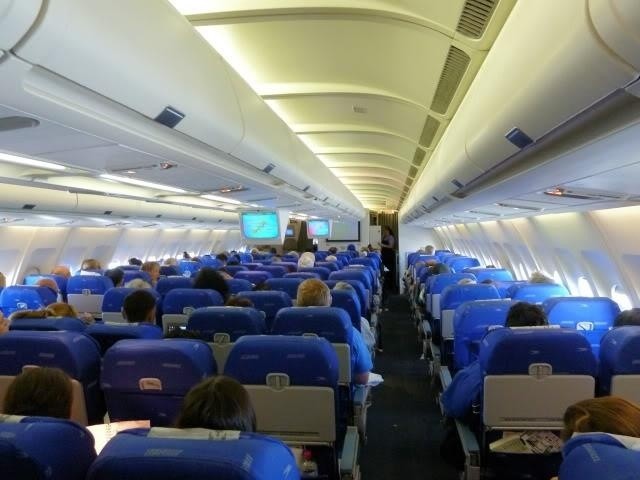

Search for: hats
xmin=298 ymin=250 xmax=315 ymax=268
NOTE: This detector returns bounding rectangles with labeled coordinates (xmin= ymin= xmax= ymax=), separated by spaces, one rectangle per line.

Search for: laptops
xmin=162 ymin=314 xmax=191 ymax=335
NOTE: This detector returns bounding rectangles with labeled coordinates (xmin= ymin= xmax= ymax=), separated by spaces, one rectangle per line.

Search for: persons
xmin=33 ymin=278 xmax=58 ymax=294
xmin=379 ymin=226 xmax=396 ymax=279
xmin=193 ymin=249 xmax=241 ymax=266
xmin=191 ymin=267 xmax=231 ymax=300
xmin=614 ymin=307 xmax=640 ymax=327
xmin=142 ymin=262 xmax=161 ymax=287
xmin=439 ymin=301 xmax=549 ymax=417
xmin=9 ymin=303 xmax=97 ymax=324
xmin=121 ymin=290 xmax=157 ymax=324
xmin=268 ymin=244 xmax=338 ymax=268
xmin=1 ymin=367 xmax=74 ymax=420
xmin=177 ymin=376 xmax=257 ymax=433
xmin=564 ymin=395 xmax=640 ymax=437
xmin=457 ymin=264 xmax=555 ymax=288
xmin=359 ymin=244 xmax=389 ymax=272
xmin=402 ymin=245 xmax=453 ymax=303
xmin=296 ymin=279 xmax=373 ymax=386
xmin=52 ymin=259 xmax=152 ymax=289
xmin=250 ymin=248 xmax=260 ymax=259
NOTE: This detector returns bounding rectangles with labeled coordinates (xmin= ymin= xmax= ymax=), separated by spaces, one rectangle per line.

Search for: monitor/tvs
xmin=307 ymin=219 xmax=333 ymax=238
xmin=239 ymin=209 xmax=289 ymax=245
xmin=285 ymin=223 xmax=300 ymax=240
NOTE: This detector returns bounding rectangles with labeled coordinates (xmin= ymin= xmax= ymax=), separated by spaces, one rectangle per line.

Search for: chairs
xmin=402 ymin=244 xmax=638 ymax=480
xmin=0 ymin=243 xmax=382 ymax=480
